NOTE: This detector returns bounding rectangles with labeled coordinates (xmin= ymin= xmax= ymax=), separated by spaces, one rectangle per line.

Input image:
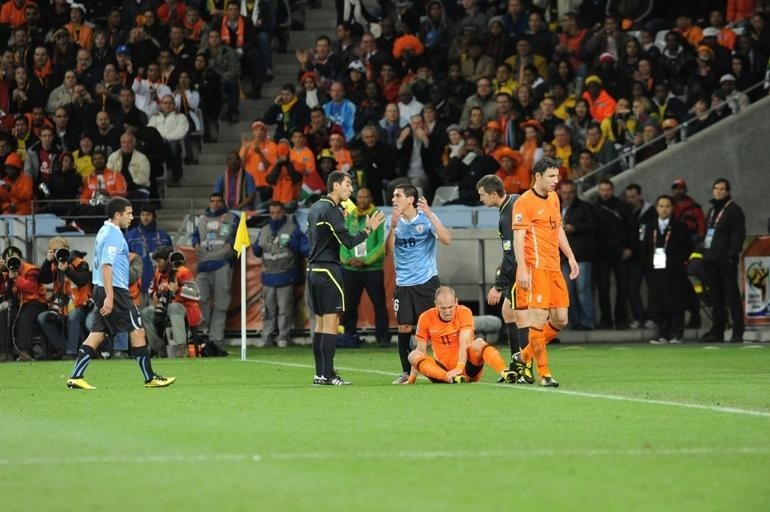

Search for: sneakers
xmin=145 ymin=376 xmax=176 ymax=388
xmin=0 ymin=345 xmax=187 ymax=362
xmin=68 ymin=376 xmax=96 ymax=389
xmin=452 ymin=375 xmax=467 ymax=384
xmin=254 ymin=338 xmax=392 ymax=348
xmin=496 ymin=352 xmax=559 ymax=387
xmin=392 ymin=373 xmax=409 ymax=384
xmin=313 ymin=375 xmax=351 ymax=387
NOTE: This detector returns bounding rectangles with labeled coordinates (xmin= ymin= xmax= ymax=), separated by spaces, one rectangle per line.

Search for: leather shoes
xmin=569 ymin=319 xmax=743 ymax=344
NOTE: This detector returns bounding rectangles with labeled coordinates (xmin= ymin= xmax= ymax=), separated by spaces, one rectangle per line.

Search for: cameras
xmin=168 ymin=251 xmax=186 ymax=268
xmin=154 ymin=290 xmax=168 ymax=326
xmin=4 ymin=248 xmax=21 ymax=272
xmin=52 ymin=247 xmax=70 ymax=264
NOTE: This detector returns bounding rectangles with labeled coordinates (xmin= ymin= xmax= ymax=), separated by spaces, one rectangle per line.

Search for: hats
xmin=484 ymin=119 xmax=546 ymax=165
xmin=671 ymin=180 xmax=685 ymax=188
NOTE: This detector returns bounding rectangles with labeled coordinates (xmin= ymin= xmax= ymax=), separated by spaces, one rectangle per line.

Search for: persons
xmin=1 ymin=0 xmax=769 ymax=388
